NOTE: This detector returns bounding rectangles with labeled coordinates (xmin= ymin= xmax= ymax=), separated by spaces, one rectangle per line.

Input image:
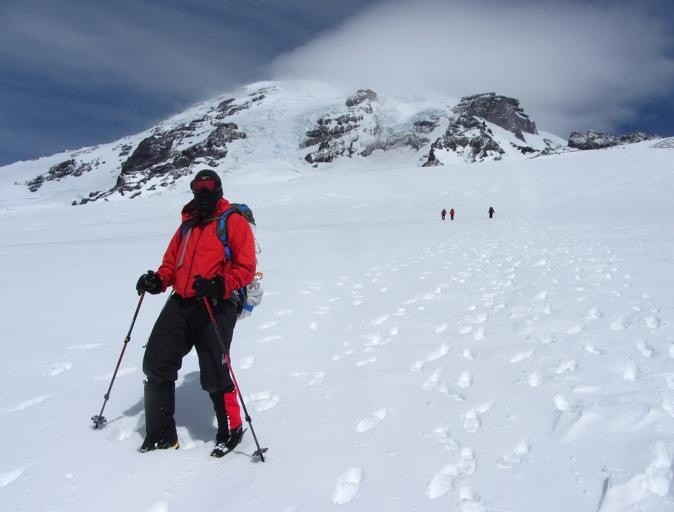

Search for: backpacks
xmin=180 ymin=202 xmax=264 ymax=319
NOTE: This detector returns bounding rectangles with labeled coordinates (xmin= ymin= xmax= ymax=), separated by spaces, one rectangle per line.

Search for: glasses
xmin=190 ymin=176 xmax=220 ymax=193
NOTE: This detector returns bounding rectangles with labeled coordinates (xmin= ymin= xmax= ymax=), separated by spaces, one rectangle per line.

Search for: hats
xmin=194 ymin=169 xmax=223 ymax=197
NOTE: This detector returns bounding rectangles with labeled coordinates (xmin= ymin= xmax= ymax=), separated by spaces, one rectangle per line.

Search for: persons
xmin=487 ymin=206 xmax=494 ymax=219
xmin=134 ymin=167 xmax=259 ymax=458
xmin=440 ymin=208 xmax=446 ymax=220
xmin=449 ymin=208 xmax=455 ymax=220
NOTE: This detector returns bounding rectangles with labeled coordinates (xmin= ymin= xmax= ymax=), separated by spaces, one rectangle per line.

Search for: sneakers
xmin=139 ymin=435 xmax=180 ymax=454
xmin=213 ymin=426 xmax=243 ymax=454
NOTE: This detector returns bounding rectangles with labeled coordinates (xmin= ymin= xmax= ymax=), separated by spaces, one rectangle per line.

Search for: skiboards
xmin=137 ymin=424 xmax=248 ymax=460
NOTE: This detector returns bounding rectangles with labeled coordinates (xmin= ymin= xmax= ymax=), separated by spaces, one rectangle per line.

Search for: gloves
xmin=192 ymin=275 xmax=225 ymax=300
xmin=136 ymin=274 xmax=162 ymax=294
